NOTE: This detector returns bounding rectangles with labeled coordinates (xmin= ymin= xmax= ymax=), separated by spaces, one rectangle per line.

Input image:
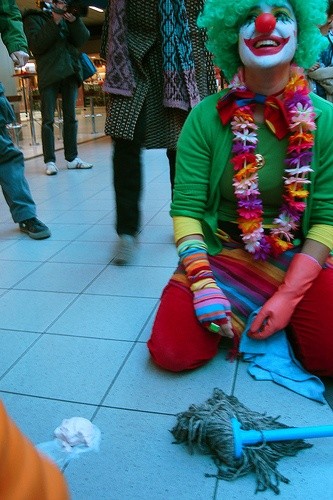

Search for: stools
xmin=6 ymin=72 xmax=104 ymax=146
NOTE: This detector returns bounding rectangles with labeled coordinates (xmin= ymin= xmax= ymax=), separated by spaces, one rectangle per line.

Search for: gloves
xmin=249 ymin=254 xmax=321 ymax=338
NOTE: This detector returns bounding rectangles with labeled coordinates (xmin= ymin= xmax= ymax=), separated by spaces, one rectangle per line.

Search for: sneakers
xmin=68 ymin=158 xmax=92 ymax=169
xmin=19 ymin=217 xmax=50 ymax=239
xmin=46 ymin=163 xmax=57 ymax=175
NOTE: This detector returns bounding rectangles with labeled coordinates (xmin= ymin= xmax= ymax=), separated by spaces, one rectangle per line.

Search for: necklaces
xmin=229 ymin=62 xmax=317 ymax=260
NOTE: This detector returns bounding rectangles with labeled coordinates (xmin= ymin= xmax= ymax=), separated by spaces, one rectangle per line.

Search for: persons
xmin=0 ymin=0 xmax=51 ymax=240
xmin=67 ymin=0 xmax=217 ymax=265
xmin=21 ymin=0 xmax=93 ymax=176
xmin=147 ymin=0 xmax=333 ymax=378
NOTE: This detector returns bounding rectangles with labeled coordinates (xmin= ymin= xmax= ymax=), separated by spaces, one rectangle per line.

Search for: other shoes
xmin=115 ymin=235 xmax=135 ymax=263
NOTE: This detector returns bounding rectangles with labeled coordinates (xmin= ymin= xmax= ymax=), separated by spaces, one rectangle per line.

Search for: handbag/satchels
xmin=81 ymin=53 xmax=96 ymax=79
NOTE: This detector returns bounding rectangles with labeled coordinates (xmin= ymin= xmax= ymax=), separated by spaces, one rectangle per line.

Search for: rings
xmin=209 ymin=321 xmax=222 ymax=333
